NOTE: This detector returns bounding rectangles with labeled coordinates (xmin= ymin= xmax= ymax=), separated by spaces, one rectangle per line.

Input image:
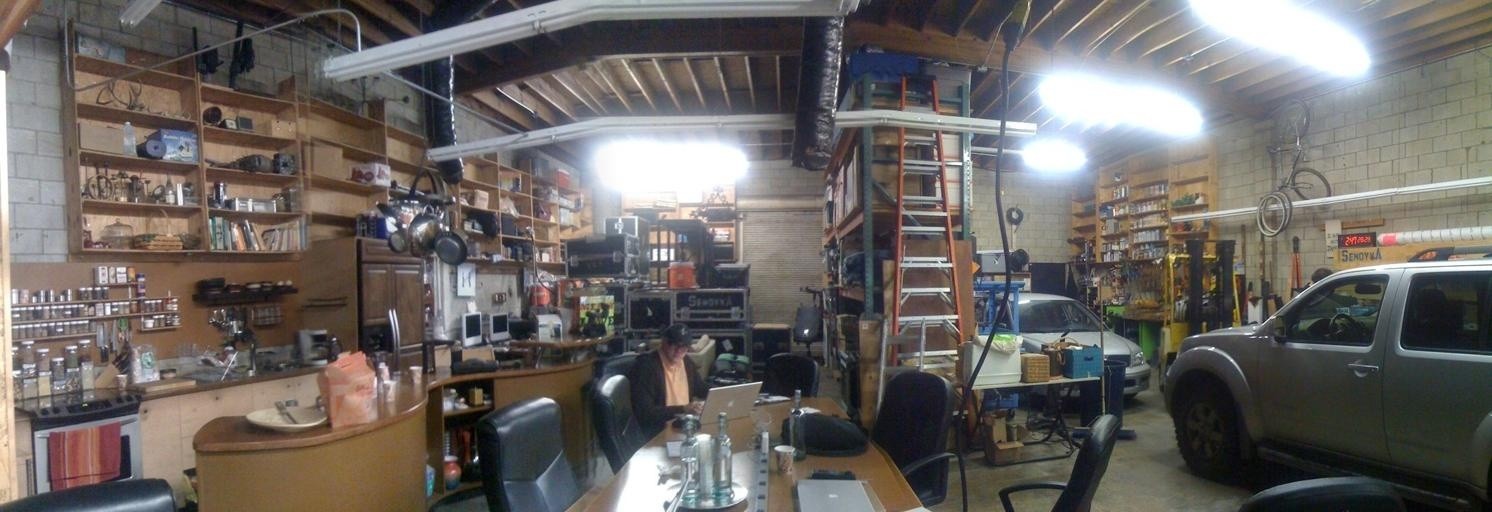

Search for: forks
xmin=275 ymin=400 xmax=297 ymax=423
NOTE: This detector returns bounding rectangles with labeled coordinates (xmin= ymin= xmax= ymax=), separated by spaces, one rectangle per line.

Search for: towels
xmin=46 ymin=422 xmax=124 ymax=495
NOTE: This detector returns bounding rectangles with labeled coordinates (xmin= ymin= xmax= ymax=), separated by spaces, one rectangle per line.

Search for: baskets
xmin=135 ymin=208 xmax=184 ymax=250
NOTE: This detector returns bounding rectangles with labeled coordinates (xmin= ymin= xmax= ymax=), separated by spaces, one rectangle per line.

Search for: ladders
xmin=891 ymin=73 xmax=975 ymax=450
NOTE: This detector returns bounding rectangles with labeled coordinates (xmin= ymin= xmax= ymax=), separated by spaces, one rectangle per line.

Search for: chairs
xmin=788 ymin=305 xmax=824 ymax=365
xmin=0 ymin=474 xmax=179 ymax=512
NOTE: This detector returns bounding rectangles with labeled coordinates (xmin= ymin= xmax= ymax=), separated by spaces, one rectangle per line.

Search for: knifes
xmin=113 ymin=351 xmax=129 ymax=373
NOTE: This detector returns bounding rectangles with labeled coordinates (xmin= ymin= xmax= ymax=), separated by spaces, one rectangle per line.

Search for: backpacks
xmin=793 ymin=305 xmax=822 ymax=344
xmin=781 ymin=412 xmax=871 ymax=457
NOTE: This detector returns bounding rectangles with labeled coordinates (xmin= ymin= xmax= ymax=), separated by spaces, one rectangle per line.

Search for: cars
xmin=973 ymin=291 xmax=1151 ymax=404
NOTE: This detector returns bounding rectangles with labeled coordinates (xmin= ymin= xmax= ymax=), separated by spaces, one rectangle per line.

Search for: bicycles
xmin=1266 ymin=97 xmax=1334 ymax=202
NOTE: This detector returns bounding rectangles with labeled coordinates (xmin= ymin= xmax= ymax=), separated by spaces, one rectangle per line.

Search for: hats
xmin=663 ymin=322 xmax=692 ymax=347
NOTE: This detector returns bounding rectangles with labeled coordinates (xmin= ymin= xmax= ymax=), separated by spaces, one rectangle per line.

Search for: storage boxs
xmin=972 ymin=248 xmax=1014 ymax=276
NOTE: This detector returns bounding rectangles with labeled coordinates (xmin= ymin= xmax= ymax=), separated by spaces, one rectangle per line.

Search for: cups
xmin=383 ymin=381 xmax=396 ymax=401
xmin=774 ymin=444 xmax=795 ymax=477
xmin=228 ymin=280 xmax=273 ymax=295
xmin=409 ymin=365 xmax=423 ymax=383
xmin=116 ymin=375 xmax=130 ymax=390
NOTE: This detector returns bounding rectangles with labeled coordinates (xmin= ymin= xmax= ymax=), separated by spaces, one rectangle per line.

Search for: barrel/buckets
xmin=528 ymin=281 xmax=553 ymax=305
xmin=665 ymin=261 xmax=697 ymax=289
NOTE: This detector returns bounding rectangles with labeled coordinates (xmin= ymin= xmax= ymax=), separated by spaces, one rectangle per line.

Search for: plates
xmin=246 ymin=406 xmax=327 ymax=431
xmin=663 ymin=481 xmax=748 ymax=509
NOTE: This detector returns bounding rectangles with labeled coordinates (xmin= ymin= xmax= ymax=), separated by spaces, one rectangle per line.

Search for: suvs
xmin=1161 ymin=257 xmax=1491 ymax=511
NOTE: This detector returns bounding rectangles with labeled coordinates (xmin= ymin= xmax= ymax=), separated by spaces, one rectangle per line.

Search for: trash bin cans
xmin=1080 ymin=358 xmax=1128 ymax=430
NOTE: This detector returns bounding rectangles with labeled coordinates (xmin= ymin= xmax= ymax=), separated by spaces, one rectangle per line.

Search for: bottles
xmin=123 ymin=119 xmax=136 ymax=157
xmin=103 ymin=218 xmax=135 ymax=249
xmin=165 ymin=183 xmax=175 ymax=205
xmin=12 ymin=286 xmax=181 ymax=339
xmin=1112 ymin=185 xmax=1128 ymax=200
xmin=787 ymin=389 xmax=808 ymax=460
xmin=100 ymin=344 xmax=109 ymax=363
xmin=444 ymin=455 xmax=462 ymax=490
xmin=331 ymin=336 xmax=340 ymax=360
xmin=136 ymin=273 xmax=146 ymax=297
xmin=213 ymin=180 xmax=227 ymax=207
xmin=678 ymin=411 xmax=735 ymax=502
xmin=127 ymin=265 xmax=136 ymax=282
xmin=426 ymin=454 xmax=436 ymax=497
xmin=450 ymin=340 xmax=462 ymax=368
xmin=274 ymin=193 xmax=286 ymax=212
xmin=377 ymin=362 xmax=390 ymax=393
xmin=13 ymin=339 xmax=96 ymax=402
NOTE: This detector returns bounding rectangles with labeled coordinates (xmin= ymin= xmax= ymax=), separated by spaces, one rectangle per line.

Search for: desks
xmin=184 ymin=331 xmax=599 ymax=512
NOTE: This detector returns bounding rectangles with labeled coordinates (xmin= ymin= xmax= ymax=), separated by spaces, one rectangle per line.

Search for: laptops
xmin=795 ymin=480 xmax=887 ymax=512
xmin=674 ymin=381 xmax=763 ymax=425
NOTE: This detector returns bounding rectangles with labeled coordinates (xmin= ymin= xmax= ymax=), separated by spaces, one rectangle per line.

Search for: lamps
xmin=114 ymin=1 xmax=162 ymax=31
xmin=319 ymin=0 xmax=863 ymax=87
xmin=417 ymin=62 xmax=1042 ymax=167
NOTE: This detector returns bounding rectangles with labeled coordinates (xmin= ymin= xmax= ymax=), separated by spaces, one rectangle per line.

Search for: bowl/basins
xmin=198 ymin=277 xmax=225 ymax=290
xmin=160 ymin=369 xmax=178 ymax=380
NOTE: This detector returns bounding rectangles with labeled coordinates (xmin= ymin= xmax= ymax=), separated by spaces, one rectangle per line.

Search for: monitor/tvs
xmin=462 ymin=312 xmax=481 ymax=347
xmin=490 ymin=312 xmax=508 ymax=341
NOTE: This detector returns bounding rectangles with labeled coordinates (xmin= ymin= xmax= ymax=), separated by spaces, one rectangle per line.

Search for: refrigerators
xmin=307 ymin=236 xmax=428 ymax=372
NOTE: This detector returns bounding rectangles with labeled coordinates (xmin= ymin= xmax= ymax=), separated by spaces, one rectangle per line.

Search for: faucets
xmin=249 ymin=339 xmax=260 ymax=373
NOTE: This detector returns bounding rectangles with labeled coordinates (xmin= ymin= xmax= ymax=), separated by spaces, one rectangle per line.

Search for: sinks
xmin=186 ymin=372 xmax=241 ymax=383
xmin=260 ymin=361 xmax=311 ymax=374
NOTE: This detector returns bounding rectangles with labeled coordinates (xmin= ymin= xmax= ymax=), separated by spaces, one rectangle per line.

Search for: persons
xmin=629 ymin=323 xmax=712 ymax=442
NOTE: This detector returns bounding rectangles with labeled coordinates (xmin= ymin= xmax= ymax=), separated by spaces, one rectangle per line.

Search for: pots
xmin=377 ymin=161 xmax=468 ymax=266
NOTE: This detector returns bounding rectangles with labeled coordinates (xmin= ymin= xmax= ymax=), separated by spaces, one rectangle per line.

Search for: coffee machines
xmin=300 ymin=329 xmax=328 ymax=365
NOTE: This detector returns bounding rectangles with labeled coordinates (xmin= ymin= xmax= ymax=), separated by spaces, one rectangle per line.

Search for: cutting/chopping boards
xmin=129 ymin=378 xmax=197 ymax=393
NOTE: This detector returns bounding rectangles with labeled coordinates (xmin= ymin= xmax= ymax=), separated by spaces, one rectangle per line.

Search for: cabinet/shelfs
xmin=618 ymin=187 xmax=738 ymax=269
xmin=138 ymin=398 xmax=187 ymax=495
xmin=1066 ymin=129 xmax=1220 ymax=306
xmin=10 ymin=280 xmax=183 ymax=344
xmin=254 ymin=371 xmax=326 ymax=417
xmin=184 ymin=383 xmax=255 ymax=479
